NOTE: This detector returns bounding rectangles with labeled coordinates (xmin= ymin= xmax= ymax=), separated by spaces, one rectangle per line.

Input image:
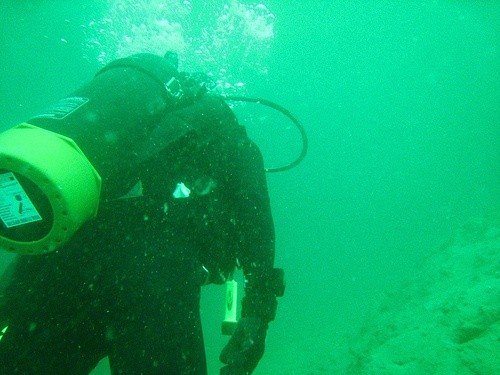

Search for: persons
xmin=0 ymin=90 xmax=286 ymax=375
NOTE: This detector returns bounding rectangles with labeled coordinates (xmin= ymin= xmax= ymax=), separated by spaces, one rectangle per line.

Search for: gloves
xmin=219 ymin=268 xmax=287 ymax=374
